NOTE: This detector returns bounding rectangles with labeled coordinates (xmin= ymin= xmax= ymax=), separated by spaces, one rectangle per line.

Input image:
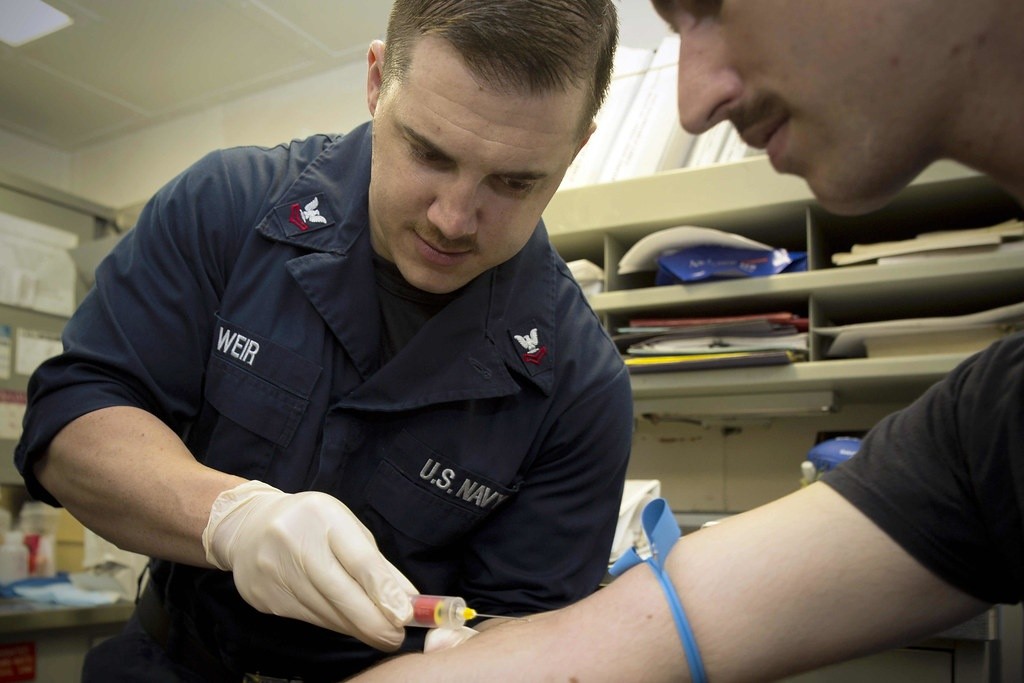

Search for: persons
xmin=13 ymin=0 xmax=634 ymax=682
xmin=349 ymin=0 xmax=1024 ymax=683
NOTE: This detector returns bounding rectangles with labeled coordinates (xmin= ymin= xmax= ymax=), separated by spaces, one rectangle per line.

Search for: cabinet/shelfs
xmin=542 ymin=150 xmax=1024 ymax=392
xmin=776 ymin=607 xmax=996 ymax=683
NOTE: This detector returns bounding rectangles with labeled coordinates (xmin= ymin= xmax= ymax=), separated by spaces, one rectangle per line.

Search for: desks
xmin=0 ymin=592 xmax=134 ymax=683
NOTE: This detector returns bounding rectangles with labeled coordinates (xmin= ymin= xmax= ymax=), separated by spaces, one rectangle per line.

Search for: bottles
xmin=1 ymin=531 xmax=29 ymax=587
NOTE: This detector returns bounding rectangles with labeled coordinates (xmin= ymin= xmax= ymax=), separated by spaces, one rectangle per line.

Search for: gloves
xmin=423 ymin=624 xmax=480 ymax=654
xmin=201 ymin=480 xmax=420 ymax=653
